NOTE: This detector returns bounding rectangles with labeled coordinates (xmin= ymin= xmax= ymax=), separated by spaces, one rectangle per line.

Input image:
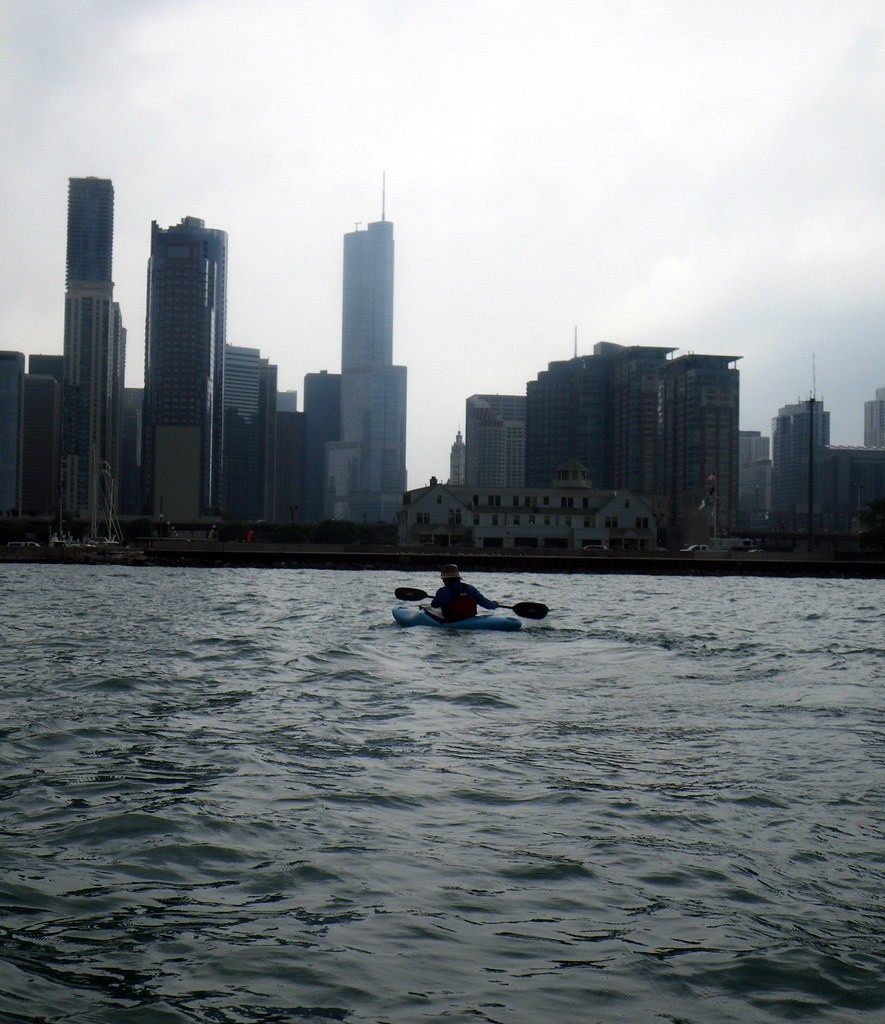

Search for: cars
xmin=583 ymin=545 xmax=607 ymax=549
xmin=7 ymin=542 xmax=40 ymax=547
xmin=679 ymin=545 xmax=710 ymax=552
xmin=748 ymin=549 xmax=765 ymax=553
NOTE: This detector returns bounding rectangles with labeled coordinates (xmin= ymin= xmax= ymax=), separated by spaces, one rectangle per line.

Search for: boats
xmin=392 ymin=606 xmax=524 ymax=634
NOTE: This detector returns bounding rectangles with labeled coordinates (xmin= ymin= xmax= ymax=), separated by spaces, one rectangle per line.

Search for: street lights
xmin=449 ymin=508 xmax=461 ymax=546
xmin=289 ymin=503 xmax=299 ymax=543
xmin=652 ymin=513 xmax=665 ymax=551
xmin=853 ymin=483 xmax=864 ymax=551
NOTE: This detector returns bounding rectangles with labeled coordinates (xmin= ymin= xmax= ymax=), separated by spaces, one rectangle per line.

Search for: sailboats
xmin=50 ymin=423 xmax=124 ymax=548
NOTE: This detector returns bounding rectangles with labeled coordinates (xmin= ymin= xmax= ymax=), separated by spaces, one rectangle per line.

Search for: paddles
xmin=395 ymin=587 xmax=549 ymax=620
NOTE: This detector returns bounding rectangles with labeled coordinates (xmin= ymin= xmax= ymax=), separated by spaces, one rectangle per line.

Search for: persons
xmin=430 ymin=563 xmax=499 ymax=622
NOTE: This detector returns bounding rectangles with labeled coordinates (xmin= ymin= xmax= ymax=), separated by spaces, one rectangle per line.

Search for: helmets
xmin=440 ymin=564 xmax=462 ymax=579
